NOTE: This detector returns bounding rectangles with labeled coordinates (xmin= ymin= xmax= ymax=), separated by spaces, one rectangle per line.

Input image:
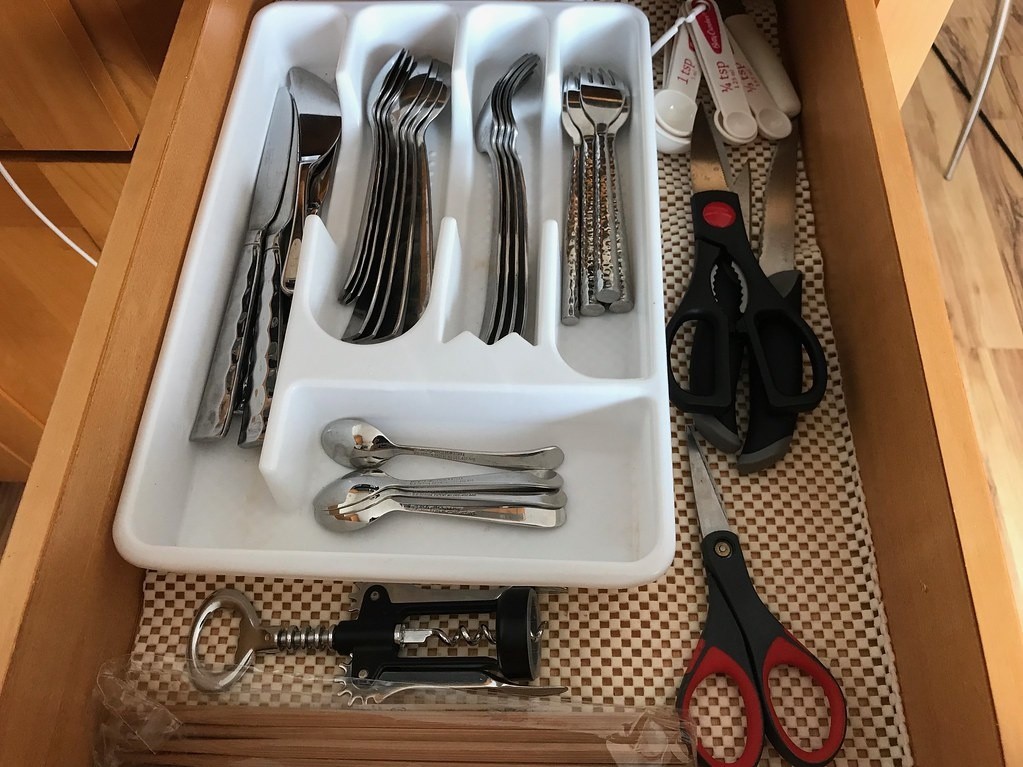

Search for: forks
xmin=560 ymin=60 xmax=632 ymax=326
xmin=471 ymin=51 xmax=540 ymax=346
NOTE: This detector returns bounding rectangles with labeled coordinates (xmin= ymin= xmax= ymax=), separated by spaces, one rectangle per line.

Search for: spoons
xmin=314 ymin=418 xmax=567 ymax=532
xmin=342 ymin=49 xmax=452 ymax=342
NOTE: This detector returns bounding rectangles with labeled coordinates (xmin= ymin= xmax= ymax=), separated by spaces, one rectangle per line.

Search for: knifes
xmin=190 ymin=63 xmax=342 ymax=448
xmin=734 ymin=113 xmax=801 ymax=472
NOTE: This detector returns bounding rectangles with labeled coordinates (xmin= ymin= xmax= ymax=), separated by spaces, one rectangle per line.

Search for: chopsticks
xmin=113 ymin=706 xmax=696 ymax=767
